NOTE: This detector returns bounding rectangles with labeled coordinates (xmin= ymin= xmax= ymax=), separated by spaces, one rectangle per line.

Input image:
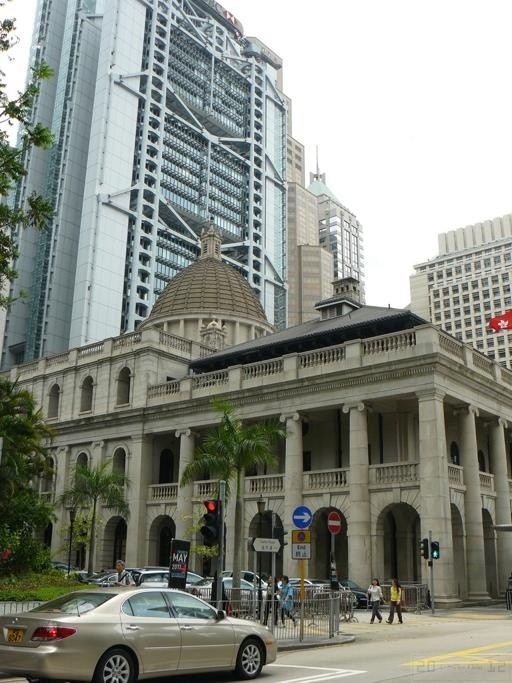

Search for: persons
xmin=263 ymin=575 xmax=280 ymax=626
xmin=365 ymin=577 xmax=385 ymax=624
xmin=113 ymin=559 xmax=136 ymax=586
xmin=209 ymin=569 xmax=231 ymax=615
xmin=385 ymin=577 xmax=404 ymax=623
xmin=277 ymin=573 xmax=300 ymax=628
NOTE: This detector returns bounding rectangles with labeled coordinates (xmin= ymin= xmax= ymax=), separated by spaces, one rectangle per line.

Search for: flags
xmin=488 ymin=309 xmax=512 ymax=331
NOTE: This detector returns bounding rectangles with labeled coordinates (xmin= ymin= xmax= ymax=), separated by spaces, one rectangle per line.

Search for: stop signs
xmin=328 ymin=512 xmax=341 ymax=535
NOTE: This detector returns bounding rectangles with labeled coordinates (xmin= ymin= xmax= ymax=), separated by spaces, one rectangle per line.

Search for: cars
xmin=0 ymin=586 xmax=280 ymax=683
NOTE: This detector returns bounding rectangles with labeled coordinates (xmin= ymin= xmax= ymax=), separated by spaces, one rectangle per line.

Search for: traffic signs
xmin=247 ymin=538 xmax=281 ymax=553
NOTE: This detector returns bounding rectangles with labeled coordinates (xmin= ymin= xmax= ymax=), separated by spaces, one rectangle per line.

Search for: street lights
xmin=257 ymin=493 xmax=267 ymax=618
xmin=67 ymin=510 xmax=77 ymax=574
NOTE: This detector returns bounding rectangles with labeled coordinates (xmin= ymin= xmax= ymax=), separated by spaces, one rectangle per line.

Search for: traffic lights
xmin=199 ymin=498 xmax=221 ymax=546
xmin=419 ymin=538 xmax=429 ymax=559
xmin=431 ymin=542 xmax=440 ymax=559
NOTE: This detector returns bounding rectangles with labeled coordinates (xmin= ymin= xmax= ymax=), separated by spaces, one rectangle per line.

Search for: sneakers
xmin=370 ymin=618 xmax=404 ymax=625
